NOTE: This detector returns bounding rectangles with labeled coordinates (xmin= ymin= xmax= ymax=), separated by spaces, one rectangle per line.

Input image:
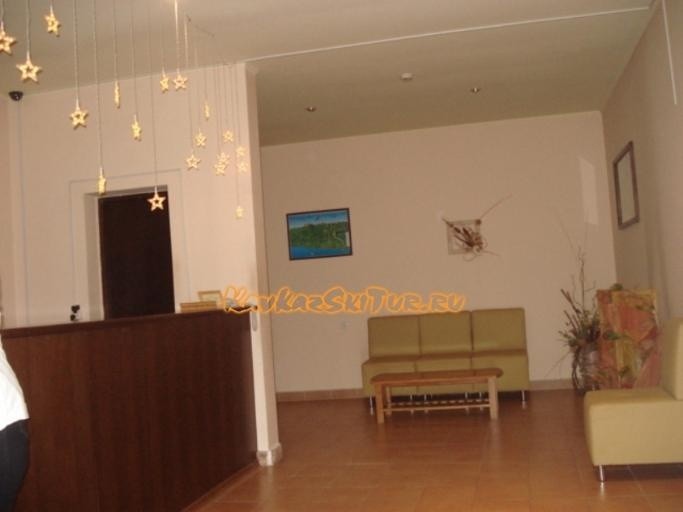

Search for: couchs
xmin=360 ymin=308 xmax=529 ymax=417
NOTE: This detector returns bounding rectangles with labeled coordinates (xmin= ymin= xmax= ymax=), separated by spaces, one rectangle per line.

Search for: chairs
xmin=581 ymin=316 xmax=682 ymax=482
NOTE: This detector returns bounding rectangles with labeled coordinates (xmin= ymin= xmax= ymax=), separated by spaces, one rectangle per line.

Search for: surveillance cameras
xmin=7 ymin=86 xmax=23 ymax=102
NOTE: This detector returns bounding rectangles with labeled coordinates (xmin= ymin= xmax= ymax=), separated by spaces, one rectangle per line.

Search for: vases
xmin=579 ymin=343 xmax=602 ymax=392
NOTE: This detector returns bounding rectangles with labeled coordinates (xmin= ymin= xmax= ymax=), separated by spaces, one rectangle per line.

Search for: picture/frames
xmin=611 ymin=139 xmax=640 ymax=230
xmin=285 ymin=207 xmax=353 ymax=261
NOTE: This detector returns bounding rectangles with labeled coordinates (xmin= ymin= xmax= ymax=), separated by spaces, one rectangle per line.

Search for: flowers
xmin=545 ymin=245 xmax=601 ymax=395
